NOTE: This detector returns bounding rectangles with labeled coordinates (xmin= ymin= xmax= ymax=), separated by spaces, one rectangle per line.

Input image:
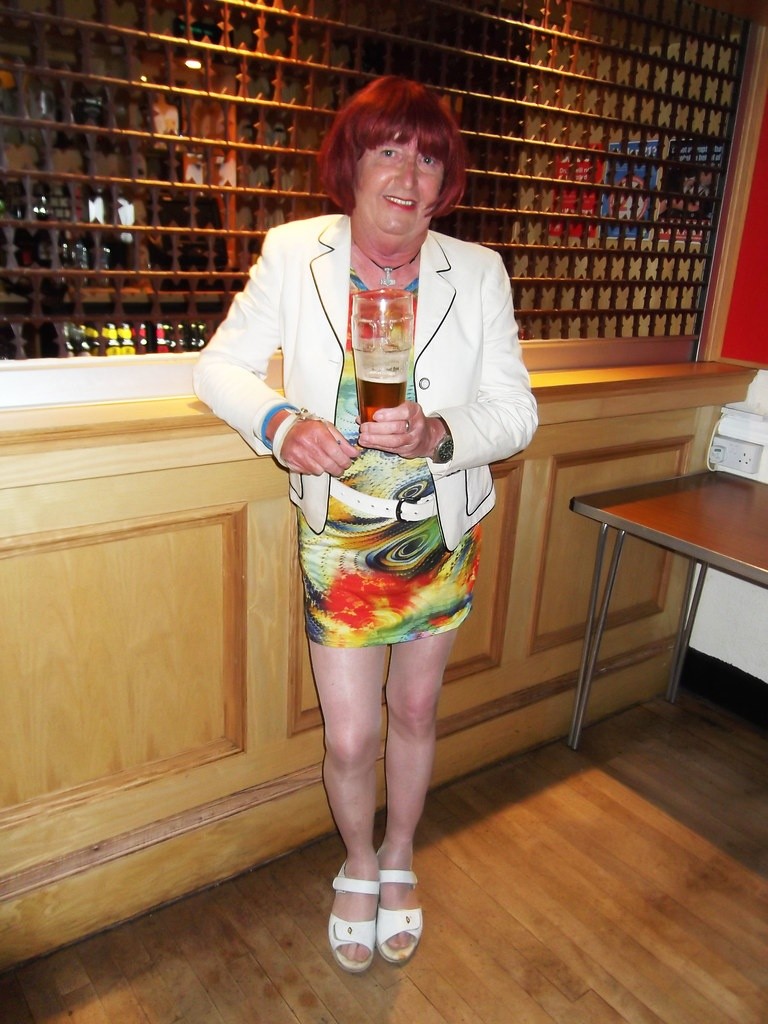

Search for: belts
xmin=330 ymin=477 xmax=438 ymax=522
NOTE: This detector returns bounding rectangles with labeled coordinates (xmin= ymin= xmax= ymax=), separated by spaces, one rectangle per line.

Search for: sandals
xmin=377 ymin=848 xmax=423 ymax=964
xmin=328 ymin=859 xmax=380 ymax=973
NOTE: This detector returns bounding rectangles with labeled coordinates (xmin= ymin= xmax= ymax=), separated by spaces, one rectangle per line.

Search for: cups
xmin=351 ymin=289 xmax=415 ymax=425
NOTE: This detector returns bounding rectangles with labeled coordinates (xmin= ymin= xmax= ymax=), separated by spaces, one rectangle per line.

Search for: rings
xmin=406 ymin=420 xmax=409 ymax=432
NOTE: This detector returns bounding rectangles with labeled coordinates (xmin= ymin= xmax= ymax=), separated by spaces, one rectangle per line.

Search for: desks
xmin=566 ymin=470 xmax=768 ymax=750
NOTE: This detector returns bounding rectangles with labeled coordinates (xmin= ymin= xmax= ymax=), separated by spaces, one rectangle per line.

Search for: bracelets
xmin=261 ymin=403 xmax=321 ymax=467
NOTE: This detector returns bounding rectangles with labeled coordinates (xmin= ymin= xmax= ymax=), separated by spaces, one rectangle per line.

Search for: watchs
xmin=433 ymin=433 xmax=454 ymax=463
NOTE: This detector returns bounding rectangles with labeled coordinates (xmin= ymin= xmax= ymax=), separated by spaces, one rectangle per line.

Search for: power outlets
xmin=710 ymin=434 xmax=763 ymax=474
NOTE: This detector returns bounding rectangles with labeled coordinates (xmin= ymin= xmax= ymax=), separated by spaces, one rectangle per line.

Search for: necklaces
xmin=352 ymin=239 xmax=422 ymax=287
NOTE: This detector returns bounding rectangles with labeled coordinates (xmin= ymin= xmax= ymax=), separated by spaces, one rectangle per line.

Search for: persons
xmin=193 ymin=75 xmax=539 ymax=973
